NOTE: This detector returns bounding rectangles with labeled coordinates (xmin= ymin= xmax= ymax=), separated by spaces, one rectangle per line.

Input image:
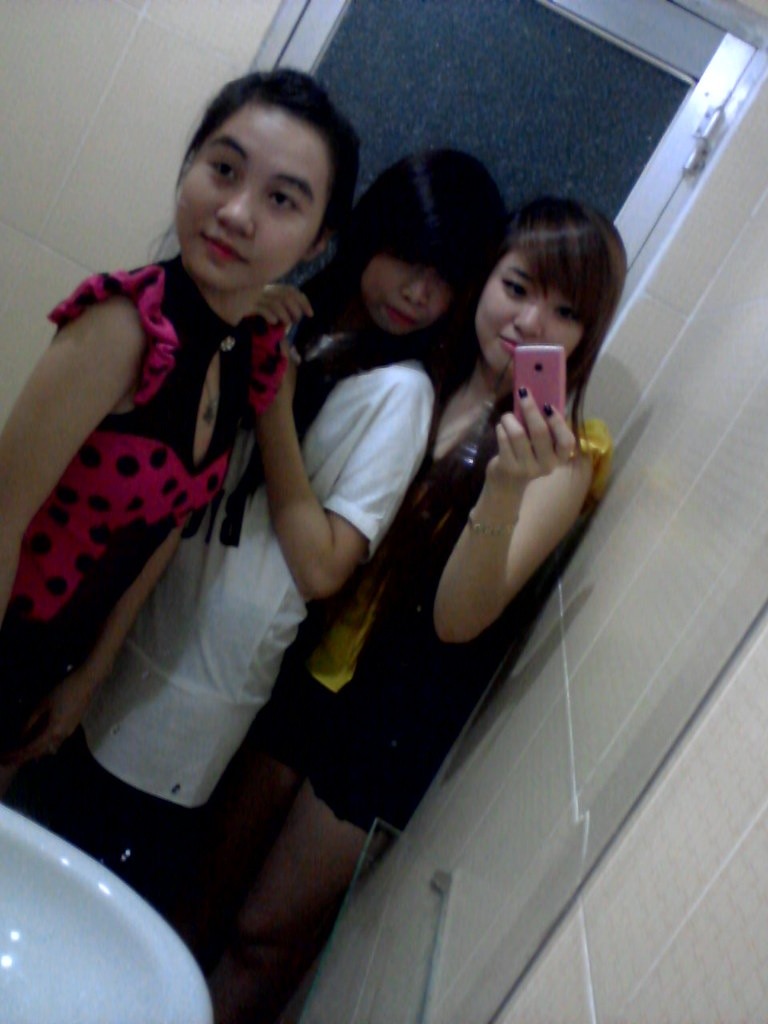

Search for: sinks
xmin=0 ymin=805 xmax=216 ymax=1024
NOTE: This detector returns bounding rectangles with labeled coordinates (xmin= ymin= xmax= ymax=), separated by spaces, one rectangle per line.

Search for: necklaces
xmin=204 ymin=377 xmax=220 ymax=424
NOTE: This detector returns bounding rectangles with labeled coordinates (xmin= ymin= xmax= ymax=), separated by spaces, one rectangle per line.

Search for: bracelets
xmin=469 ymin=509 xmax=519 ymax=536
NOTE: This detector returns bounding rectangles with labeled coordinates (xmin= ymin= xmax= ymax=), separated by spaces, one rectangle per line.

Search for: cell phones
xmin=512 ymin=346 xmax=570 ymax=442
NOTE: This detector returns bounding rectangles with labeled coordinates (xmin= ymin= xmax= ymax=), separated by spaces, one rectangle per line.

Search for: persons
xmin=0 ymin=67 xmax=364 ymax=809
xmin=167 ymin=190 xmax=628 ymax=1024
xmin=11 ymin=147 xmax=510 ymax=880
